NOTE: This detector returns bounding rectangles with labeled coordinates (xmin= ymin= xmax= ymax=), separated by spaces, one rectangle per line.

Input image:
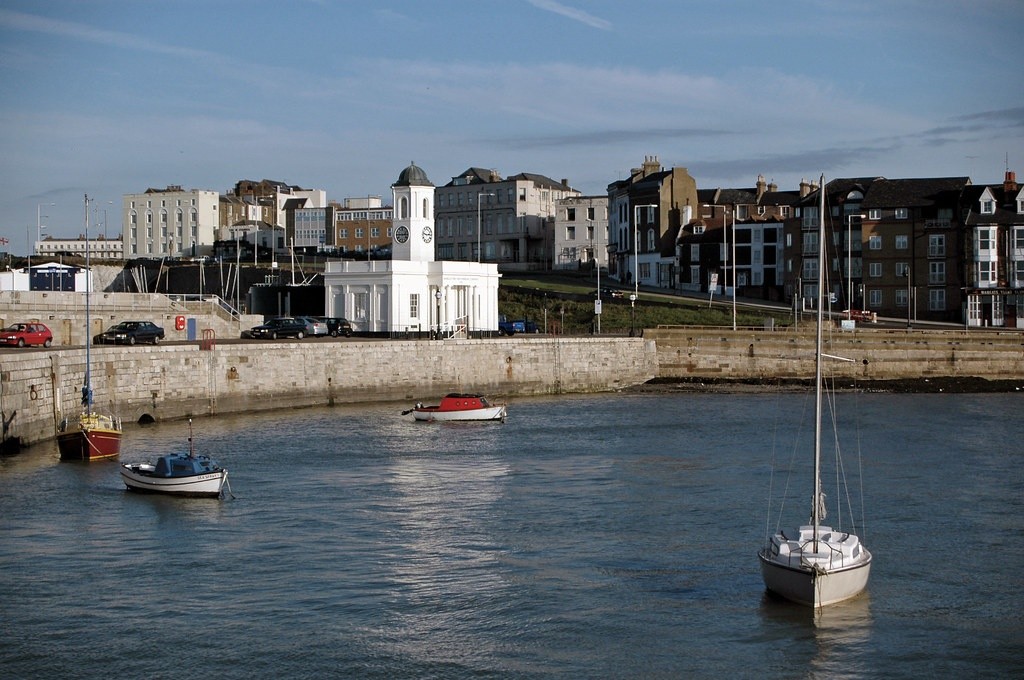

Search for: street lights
xmin=584 ymin=219 xmax=594 ymax=280
xmin=633 ymin=203 xmax=660 ymax=298
xmin=5 ymin=264 xmax=16 ymax=313
xmin=169 ymin=236 xmax=173 ymax=260
xmin=723 ymin=203 xmax=762 ymax=334
xmin=699 ymin=203 xmax=729 ymax=299
xmin=255 ymin=195 xmax=275 ymax=266
xmin=476 ymin=192 xmax=497 ymax=263
xmin=847 ymin=212 xmax=867 ymax=321
xmin=595 ymin=256 xmax=601 ymax=335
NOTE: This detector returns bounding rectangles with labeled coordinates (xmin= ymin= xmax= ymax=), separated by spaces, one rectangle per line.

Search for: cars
xmin=294 ymin=316 xmax=329 ymax=337
xmin=250 ymin=317 xmax=309 ymax=340
xmin=588 ymin=286 xmax=625 ymax=299
xmin=0 ymin=323 xmax=53 ymax=349
xmin=317 ymin=318 xmax=354 ymax=338
xmin=93 ymin=321 xmax=166 ymax=345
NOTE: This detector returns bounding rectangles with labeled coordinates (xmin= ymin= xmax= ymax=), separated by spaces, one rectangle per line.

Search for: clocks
xmin=394 ymin=225 xmax=411 ymax=245
xmin=422 ymin=226 xmax=432 ymax=244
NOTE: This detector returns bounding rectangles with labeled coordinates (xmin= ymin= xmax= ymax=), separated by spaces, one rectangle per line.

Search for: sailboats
xmin=57 ymin=193 xmax=120 ymax=464
xmin=760 ymin=174 xmax=874 ymax=608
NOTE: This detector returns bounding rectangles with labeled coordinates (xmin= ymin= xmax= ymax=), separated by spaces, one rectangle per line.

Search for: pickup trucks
xmin=499 ymin=320 xmax=525 ymax=336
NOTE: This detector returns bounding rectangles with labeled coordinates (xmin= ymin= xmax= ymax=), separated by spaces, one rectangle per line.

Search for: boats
xmin=121 ymin=413 xmax=227 ymax=498
xmin=401 ymin=391 xmax=507 ymax=421
